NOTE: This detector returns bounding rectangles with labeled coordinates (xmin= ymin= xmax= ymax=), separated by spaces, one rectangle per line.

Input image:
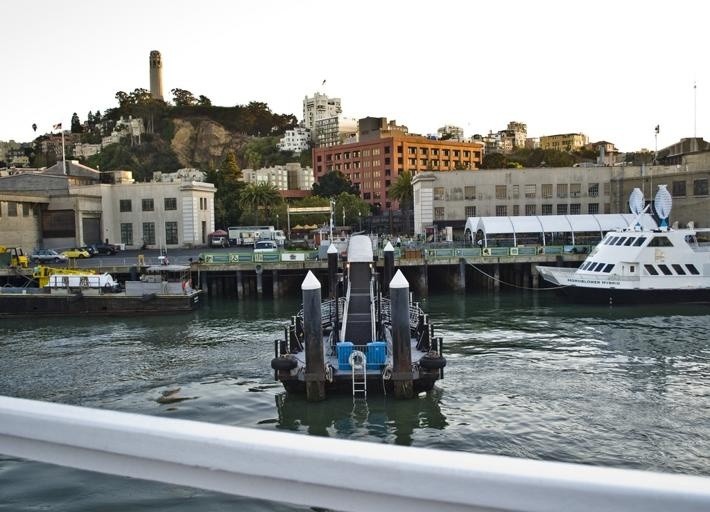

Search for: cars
xmin=211 ymin=236 xmax=226 ymax=248
xmin=29 ymin=243 xmax=121 ymax=264
xmin=253 ymin=240 xmax=278 ymax=256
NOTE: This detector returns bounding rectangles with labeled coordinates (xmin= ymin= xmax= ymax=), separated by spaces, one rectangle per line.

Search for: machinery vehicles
xmin=0 ymin=247 xmax=27 ymax=268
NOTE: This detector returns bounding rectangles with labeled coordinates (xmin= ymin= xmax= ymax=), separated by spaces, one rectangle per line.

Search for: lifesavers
xmin=349 ymin=351 xmax=366 ymax=369
xmin=189 ymin=279 xmax=192 ymax=286
xmin=182 ymin=281 xmax=186 ymax=289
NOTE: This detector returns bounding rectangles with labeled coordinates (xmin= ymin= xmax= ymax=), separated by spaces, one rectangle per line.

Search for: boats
xmin=0 ymin=265 xmax=202 ymax=313
xmin=270 ymin=234 xmax=446 ymax=400
xmin=534 ymin=228 xmax=710 ymax=305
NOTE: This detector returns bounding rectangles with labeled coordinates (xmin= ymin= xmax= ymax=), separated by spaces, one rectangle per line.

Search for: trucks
xmin=228 ymin=226 xmax=287 ymax=245
